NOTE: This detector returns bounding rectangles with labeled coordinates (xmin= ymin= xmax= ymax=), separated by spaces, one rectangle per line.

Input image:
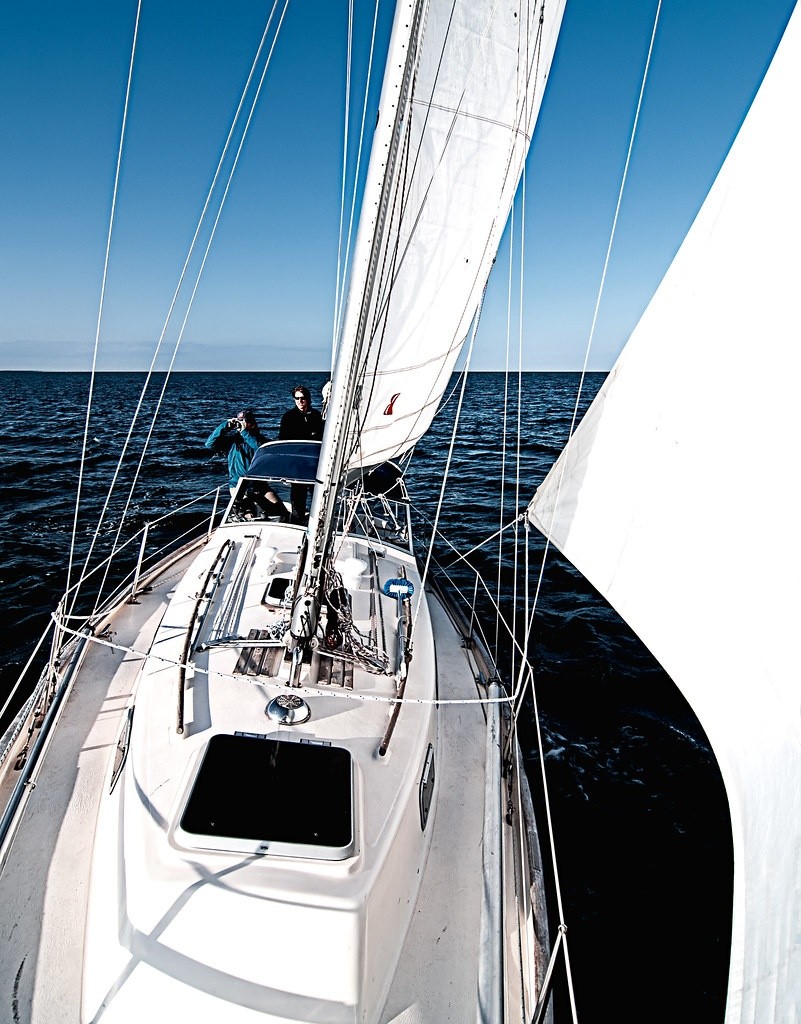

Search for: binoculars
xmin=226 ymin=419 xmax=244 ymax=430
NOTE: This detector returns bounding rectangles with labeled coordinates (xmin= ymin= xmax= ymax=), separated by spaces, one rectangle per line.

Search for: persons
xmin=205 ymin=404 xmax=287 ymax=520
xmin=279 ymin=376 xmax=331 ymax=525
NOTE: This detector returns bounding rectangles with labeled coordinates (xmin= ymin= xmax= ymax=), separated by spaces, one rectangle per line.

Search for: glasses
xmin=294 ymin=397 xmax=306 ymax=400
